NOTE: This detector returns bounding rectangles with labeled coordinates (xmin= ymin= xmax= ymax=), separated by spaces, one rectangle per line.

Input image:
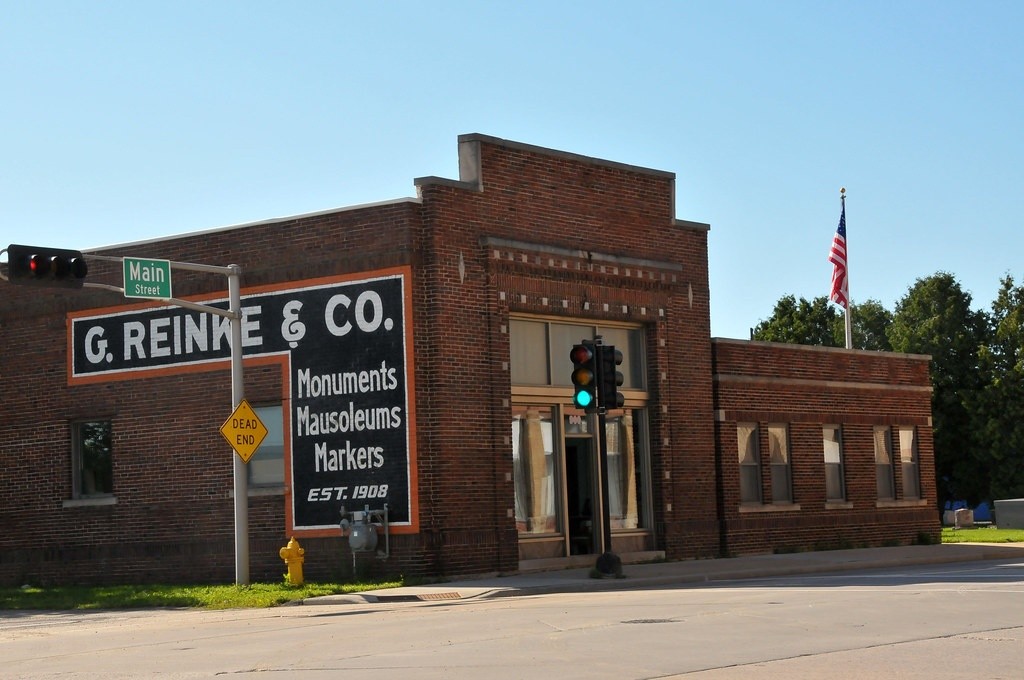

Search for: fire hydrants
xmin=279 ymin=536 xmax=306 ymax=586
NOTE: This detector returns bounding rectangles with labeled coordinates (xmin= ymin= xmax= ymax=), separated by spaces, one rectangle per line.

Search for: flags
xmin=830 ymin=203 xmax=847 ymax=310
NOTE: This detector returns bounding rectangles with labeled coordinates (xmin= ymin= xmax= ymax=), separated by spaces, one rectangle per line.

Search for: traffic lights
xmin=569 ymin=343 xmax=596 ymax=410
xmin=601 ymin=345 xmax=624 ymax=409
xmin=8 ymin=244 xmax=88 ymax=290
